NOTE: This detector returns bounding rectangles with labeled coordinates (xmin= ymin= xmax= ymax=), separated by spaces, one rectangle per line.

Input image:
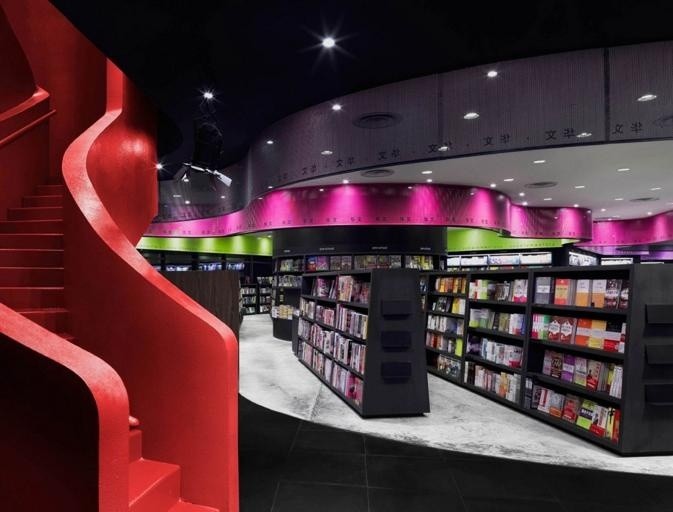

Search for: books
xmin=240 ymin=276 xmax=272 ymax=314
xmin=296 ymin=276 xmax=371 ymax=411
xmin=301 ymin=257 xmax=444 ymax=271
xmin=419 ymin=274 xmax=629 ymax=444
xmin=446 ymin=265 xmax=550 ymax=272
xmin=271 ymin=259 xmax=302 ymax=320
